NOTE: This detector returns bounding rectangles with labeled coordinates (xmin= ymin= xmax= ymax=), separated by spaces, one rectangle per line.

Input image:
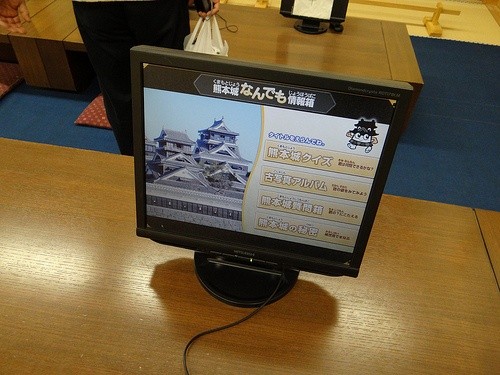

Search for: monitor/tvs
xmin=130 ymin=45 xmax=414 ymax=309
xmin=280 ymin=0 xmax=349 ymax=34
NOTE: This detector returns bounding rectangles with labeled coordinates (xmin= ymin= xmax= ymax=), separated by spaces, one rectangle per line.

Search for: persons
xmin=71 ymin=0 xmax=220 ymax=154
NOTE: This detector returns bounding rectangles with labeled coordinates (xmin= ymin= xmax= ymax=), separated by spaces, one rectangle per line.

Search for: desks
xmin=4 ymin=2 xmax=424 ymax=134
xmin=0 ymin=140 xmax=500 ymax=375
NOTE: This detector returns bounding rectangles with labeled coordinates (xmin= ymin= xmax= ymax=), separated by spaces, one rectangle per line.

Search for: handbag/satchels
xmin=183 ymin=12 xmax=228 ymax=56
